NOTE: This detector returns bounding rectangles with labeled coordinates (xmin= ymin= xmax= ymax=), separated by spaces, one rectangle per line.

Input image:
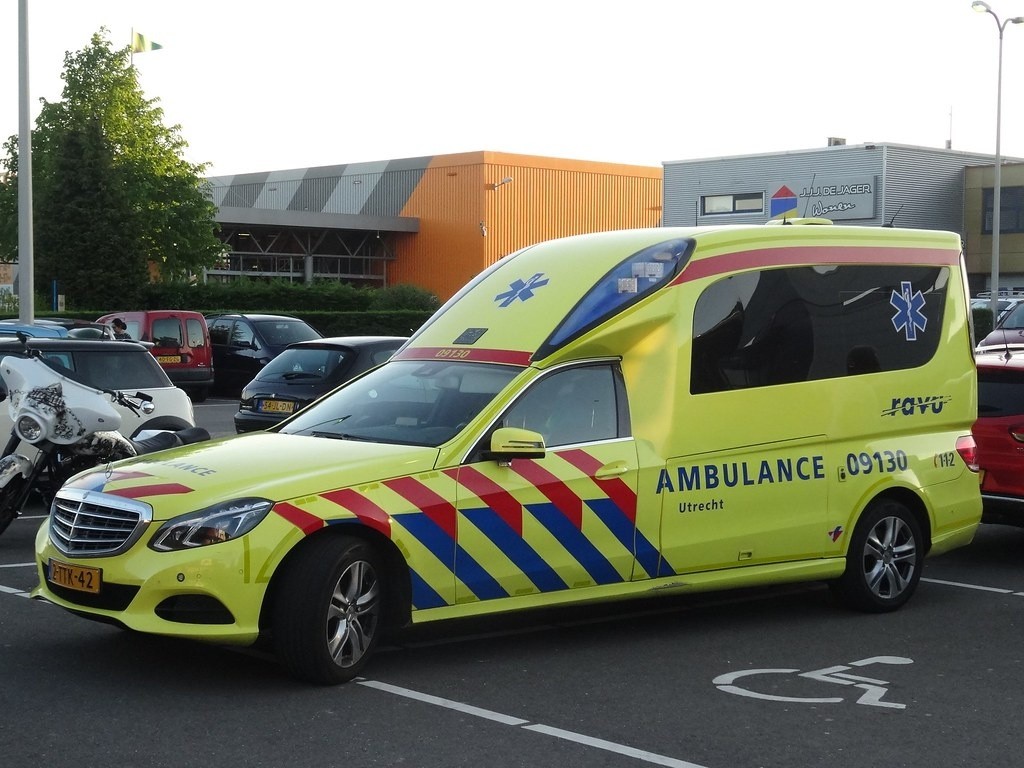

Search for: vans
xmin=93 ymin=310 xmax=214 ymax=403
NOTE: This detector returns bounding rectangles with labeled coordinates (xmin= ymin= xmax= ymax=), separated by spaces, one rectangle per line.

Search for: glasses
xmin=111 ymin=326 xmax=116 ymax=329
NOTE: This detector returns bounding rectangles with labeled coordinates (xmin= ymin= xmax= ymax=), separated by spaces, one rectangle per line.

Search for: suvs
xmin=202 ymin=311 xmax=337 ymax=392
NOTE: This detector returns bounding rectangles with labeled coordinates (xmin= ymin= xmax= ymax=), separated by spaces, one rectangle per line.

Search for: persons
xmin=112 ymin=319 xmax=130 ymax=339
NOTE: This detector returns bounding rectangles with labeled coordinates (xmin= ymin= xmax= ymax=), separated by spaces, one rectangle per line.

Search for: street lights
xmin=970 ymin=0 xmax=1024 ymax=333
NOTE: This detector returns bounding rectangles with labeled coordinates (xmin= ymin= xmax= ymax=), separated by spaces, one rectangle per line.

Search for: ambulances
xmin=28 ymin=216 xmax=987 ymax=690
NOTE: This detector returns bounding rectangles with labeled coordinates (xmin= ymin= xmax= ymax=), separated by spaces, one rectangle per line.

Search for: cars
xmin=968 ymin=290 xmax=1024 ymax=526
xmin=234 ymin=335 xmax=440 ymax=435
xmin=0 ymin=318 xmax=198 ymax=505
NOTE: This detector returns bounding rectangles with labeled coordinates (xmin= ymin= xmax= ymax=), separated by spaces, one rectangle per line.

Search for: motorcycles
xmin=0 ymin=328 xmax=211 ymax=538
